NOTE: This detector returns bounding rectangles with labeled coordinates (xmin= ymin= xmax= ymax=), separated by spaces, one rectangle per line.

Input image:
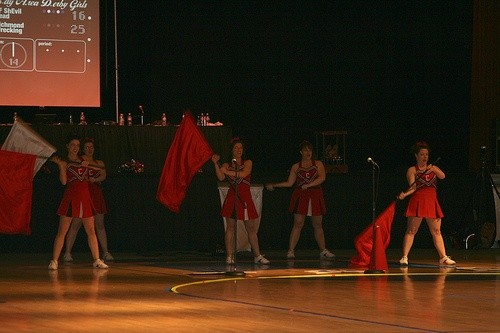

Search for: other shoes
xmin=254 ymin=255 xmax=270 ymax=264
xmin=288 ymin=248 xmax=295 ymax=258
xmin=400 ymin=256 xmax=408 ymax=265
xmin=440 ymin=256 xmax=456 ymax=265
xmin=103 ymin=255 xmax=114 ymax=261
xmin=225 ymin=256 xmax=235 ymax=264
xmin=47 ymin=260 xmax=58 ymax=270
xmin=93 ymin=259 xmax=110 ymax=269
xmin=319 ymin=249 xmax=335 ymax=258
xmin=63 ymin=255 xmax=73 ymax=262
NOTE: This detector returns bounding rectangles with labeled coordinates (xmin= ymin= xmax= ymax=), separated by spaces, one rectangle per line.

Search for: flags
xmin=348 ymin=202 xmax=395 ymax=269
xmin=1 ymin=117 xmax=55 ymax=179
xmin=156 ymin=113 xmax=212 ymax=214
xmin=0 ymin=150 xmax=37 ymax=234
xmin=218 ymin=185 xmax=264 ymax=253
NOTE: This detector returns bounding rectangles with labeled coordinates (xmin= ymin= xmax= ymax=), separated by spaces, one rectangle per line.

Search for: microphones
xmin=368 ymin=158 xmax=378 ymax=167
xmin=481 ymin=146 xmax=487 ymax=159
xmin=232 ymin=159 xmax=237 ymax=164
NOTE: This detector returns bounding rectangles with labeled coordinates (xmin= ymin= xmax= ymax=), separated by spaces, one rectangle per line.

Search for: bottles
xmin=13 ymin=113 xmax=18 ymax=124
xmin=128 ymin=113 xmax=132 ymax=126
xmin=68 ymin=112 xmax=72 ymax=125
xmin=200 ymin=113 xmax=209 ymax=126
xmin=80 ymin=112 xmax=85 ymax=125
xmin=119 ymin=114 xmax=124 ymax=126
xmin=197 ymin=116 xmax=200 ymax=126
xmin=162 ymin=113 xmax=166 ymax=126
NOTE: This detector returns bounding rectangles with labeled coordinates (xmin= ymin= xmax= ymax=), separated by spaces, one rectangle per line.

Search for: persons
xmin=397 ymin=141 xmax=455 ymax=265
xmin=63 ymin=136 xmax=114 ymax=262
xmin=49 ymin=133 xmax=108 ymax=269
xmin=211 ymin=137 xmax=271 ymax=264
xmin=268 ymin=140 xmax=336 ymax=258
